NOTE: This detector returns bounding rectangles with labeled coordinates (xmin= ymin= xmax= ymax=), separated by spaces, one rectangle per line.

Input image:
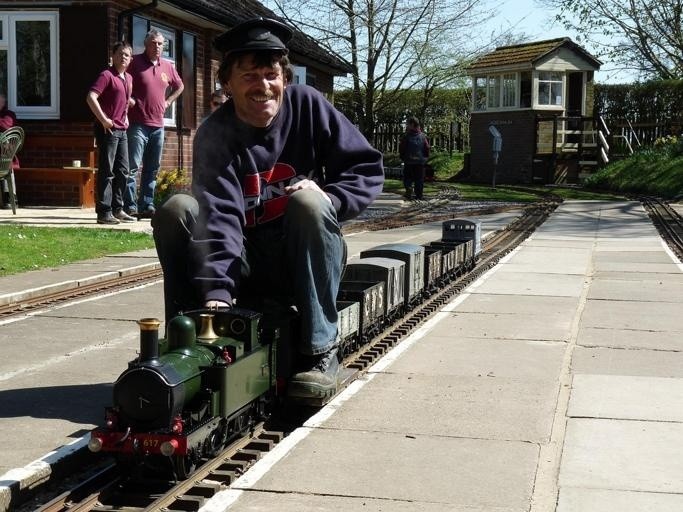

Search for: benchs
xmin=13 ymin=135 xmax=100 ymax=209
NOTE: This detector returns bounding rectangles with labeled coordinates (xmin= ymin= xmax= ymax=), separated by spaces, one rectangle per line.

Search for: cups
xmin=72 ymin=160 xmax=81 ymax=169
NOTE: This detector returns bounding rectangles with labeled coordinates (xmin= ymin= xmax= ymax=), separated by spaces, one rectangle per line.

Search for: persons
xmin=85 ymin=41 xmax=137 ymax=226
xmin=151 ymin=18 xmax=387 ymax=410
xmin=0 ymin=92 xmax=20 ymax=209
xmin=199 ymin=89 xmax=230 ymax=125
xmin=538 ymin=83 xmax=555 ymax=105
xmin=121 ymin=30 xmax=185 ymax=220
xmin=397 ymin=115 xmax=430 ymax=201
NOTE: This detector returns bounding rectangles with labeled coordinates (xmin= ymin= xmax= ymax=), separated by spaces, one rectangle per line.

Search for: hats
xmin=215 ymin=16 xmax=293 ymax=54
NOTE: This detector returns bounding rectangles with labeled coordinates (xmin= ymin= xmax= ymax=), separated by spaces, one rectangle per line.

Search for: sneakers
xmin=406 ymin=187 xmax=424 ymax=200
xmin=288 ymin=347 xmax=342 ymax=396
xmin=96 ymin=208 xmax=156 ymax=225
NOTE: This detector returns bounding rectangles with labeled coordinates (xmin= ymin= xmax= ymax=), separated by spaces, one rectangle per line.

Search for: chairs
xmin=0 ymin=126 xmax=25 ymax=214
